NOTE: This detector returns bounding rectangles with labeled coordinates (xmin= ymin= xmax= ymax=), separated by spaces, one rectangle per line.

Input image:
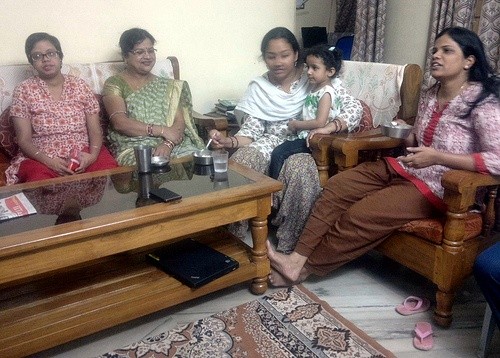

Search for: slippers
xmin=414 ymin=322 xmax=434 ymax=351
xmin=396 ymin=295 xmax=430 ymax=316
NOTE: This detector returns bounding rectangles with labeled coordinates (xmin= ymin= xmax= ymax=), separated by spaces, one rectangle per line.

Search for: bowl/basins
xmin=380 ymin=121 xmax=413 ymax=139
xmin=192 ymin=150 xmax=213 ymax=165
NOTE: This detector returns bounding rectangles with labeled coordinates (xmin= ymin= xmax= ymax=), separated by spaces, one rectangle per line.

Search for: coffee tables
xmin=0 ymin=155 xmax=284 ymax=358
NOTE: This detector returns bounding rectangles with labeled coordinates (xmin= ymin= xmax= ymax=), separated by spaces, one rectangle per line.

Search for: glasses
xmin=127 ymin=49 xmax=157 ymax=56
xmin=30 ymin=52 xmax=59 ymax=61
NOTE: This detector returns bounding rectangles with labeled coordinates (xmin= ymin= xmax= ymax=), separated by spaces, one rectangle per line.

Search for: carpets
xmin=92 ymin=283 xmax=398 ymax=358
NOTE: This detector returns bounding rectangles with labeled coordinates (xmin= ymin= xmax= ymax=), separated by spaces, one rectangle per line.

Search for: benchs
xmin=0 ymin=56 xmax=228 ymax=187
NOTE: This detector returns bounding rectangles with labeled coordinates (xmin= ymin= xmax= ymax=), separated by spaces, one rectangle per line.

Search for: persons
xmin=101 ymin=28 xmax=204 ymax=168
xmin=206 ymin=27 xmax=364 ymax=255
xmin=270 ymin=44 xmax=342 ymax=181
xmin=5 ymin=33 xmax=117 ymax=185
xmin=266 ymin=26 xmax=500 ymax=287
xmin=24 ymin=176 xmax=107 ymax=226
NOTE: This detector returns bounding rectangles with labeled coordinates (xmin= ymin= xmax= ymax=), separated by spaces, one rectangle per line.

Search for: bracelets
xmin=164 ymin=139 xmax=174 ymax=150
xmin=146 ymin=124 xmax=164 ymax=137
xmin=32 ymin=151 xmax=40 ymax=157
xmin=333 ymin=119 xmax=342 ymax=133
xmin=229 ymin=136 xmax=239 ymax=149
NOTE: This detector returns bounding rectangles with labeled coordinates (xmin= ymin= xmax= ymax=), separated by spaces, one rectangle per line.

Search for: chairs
xmin=310 ymin=59 xmax=423 ymax=189
xmin=331 ymin=134 xmax=500 ymax=329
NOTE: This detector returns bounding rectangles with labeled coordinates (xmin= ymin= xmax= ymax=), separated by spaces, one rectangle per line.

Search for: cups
xmin=134 ymin=145 xmax=152 ymax=173
xmin=150 ymin=144 xmax=171 ymax=167
xmin=212 ymin=150 xmax=229 ymax=173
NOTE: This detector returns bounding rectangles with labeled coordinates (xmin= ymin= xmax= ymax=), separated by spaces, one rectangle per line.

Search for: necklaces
xmin=276 ymin=79 xmax=300 ymax=94
